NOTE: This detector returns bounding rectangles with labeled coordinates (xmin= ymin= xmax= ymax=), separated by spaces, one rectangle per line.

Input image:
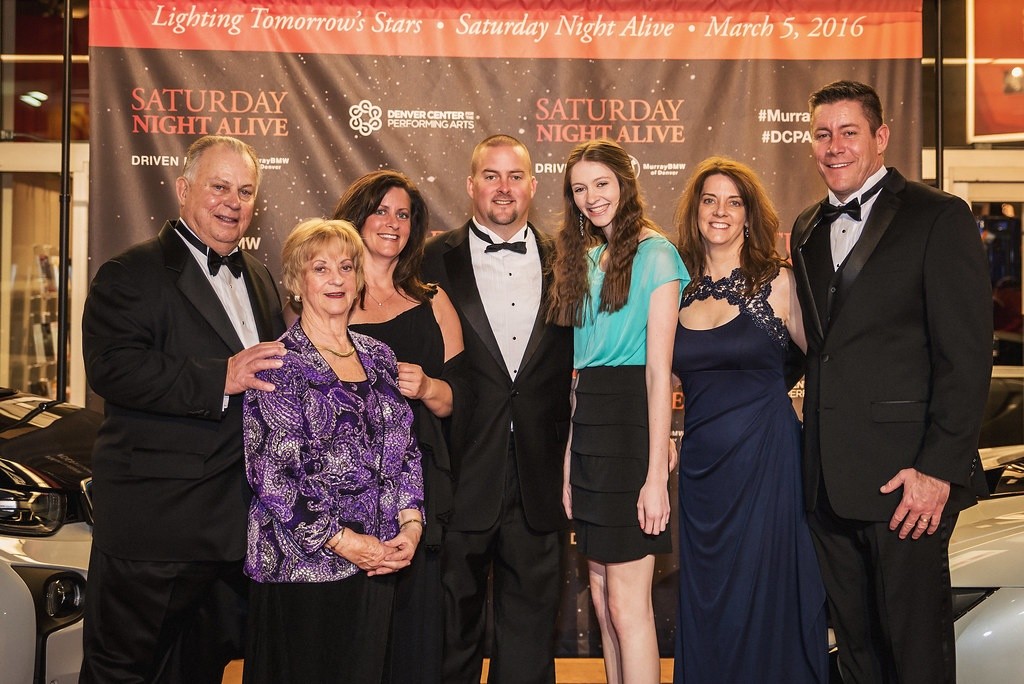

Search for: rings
xmin=919 ymin=517 xmax=930 ymax=523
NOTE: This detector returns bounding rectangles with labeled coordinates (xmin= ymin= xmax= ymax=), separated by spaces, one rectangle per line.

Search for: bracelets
xmin=399 ymin=519 xmax=424 ymax=530
xmin=330 ymin=526 xmax=345 ymax=550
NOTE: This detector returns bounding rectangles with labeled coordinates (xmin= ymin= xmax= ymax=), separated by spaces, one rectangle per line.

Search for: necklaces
xmin=309 ymin=342 xmax=356 ymax=357
xmin=362 ymin=283 xmax=399 ymax=306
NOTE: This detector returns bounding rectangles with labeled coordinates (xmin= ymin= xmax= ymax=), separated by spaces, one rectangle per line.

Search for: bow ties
xmin=175 ymin=220 xmax=244 ymax=279
xmin=820 ymin=172 xmax=890 ymax=225
xmin=469 ymin=217 xmax=528 ymax=254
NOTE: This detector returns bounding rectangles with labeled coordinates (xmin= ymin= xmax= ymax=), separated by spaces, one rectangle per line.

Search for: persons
xmin=670 ymin=156 xmax=830 ymax=684
xmin=789 ymin=78 xmax=995 ymax=684
xmin=77 ymin=133 xmax=691 ymax=684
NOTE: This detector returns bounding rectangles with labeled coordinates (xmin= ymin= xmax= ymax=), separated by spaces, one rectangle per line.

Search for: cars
xmin=946 ymin=365 xmax=1024 ymax=684
xmin=0 ymin=387 xmax=112 ymax=684
